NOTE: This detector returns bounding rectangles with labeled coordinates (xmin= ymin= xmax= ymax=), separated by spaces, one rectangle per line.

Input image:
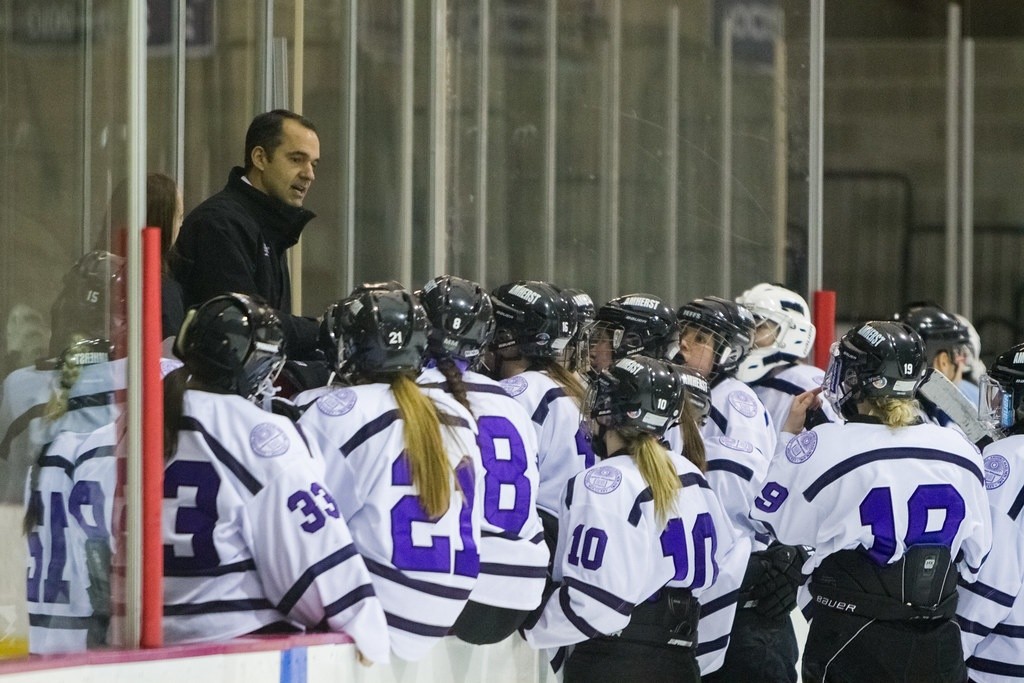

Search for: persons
xmin=50 ymin=171 xmax=185 ymax=364
xmin=168 ymin=110 xmax=320 ymax=314
xmin=2 ymin=252 xmax=1024 ymax=682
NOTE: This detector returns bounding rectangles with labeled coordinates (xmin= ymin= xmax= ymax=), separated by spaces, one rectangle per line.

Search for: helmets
xmin=414 ymin=276 xmax=493 ymax=365
xmin=890 ymin=306 xmax=972 ymax=364
xmin=578 ymin=354 xmax=683 ymax=456
xmin=557 ymin=286 xmax=594 ymax=324
xmin=733 ymin=280 xmax=816 ymax=383
xmin=579 ymin=294 xmax=677 ymax=378
xmin=51 ymin=250 xmax=126 ymax=339
xmin=173 ymin=295 xmax=285 ymax=393
xmin=492 ymin=279 xmax=577 ymax=359
xmin=674 ymin=298 xmax=758 ymax=382
xmin=320 ymin=282 xmax=429 ymax=379
xmin=821 ymin=320 xmax=930 ymax=425
xmin=986 ymin=342 xmax=1024 ymax=435
xmin=670 ymin=367 xmax=712 ymax=423
